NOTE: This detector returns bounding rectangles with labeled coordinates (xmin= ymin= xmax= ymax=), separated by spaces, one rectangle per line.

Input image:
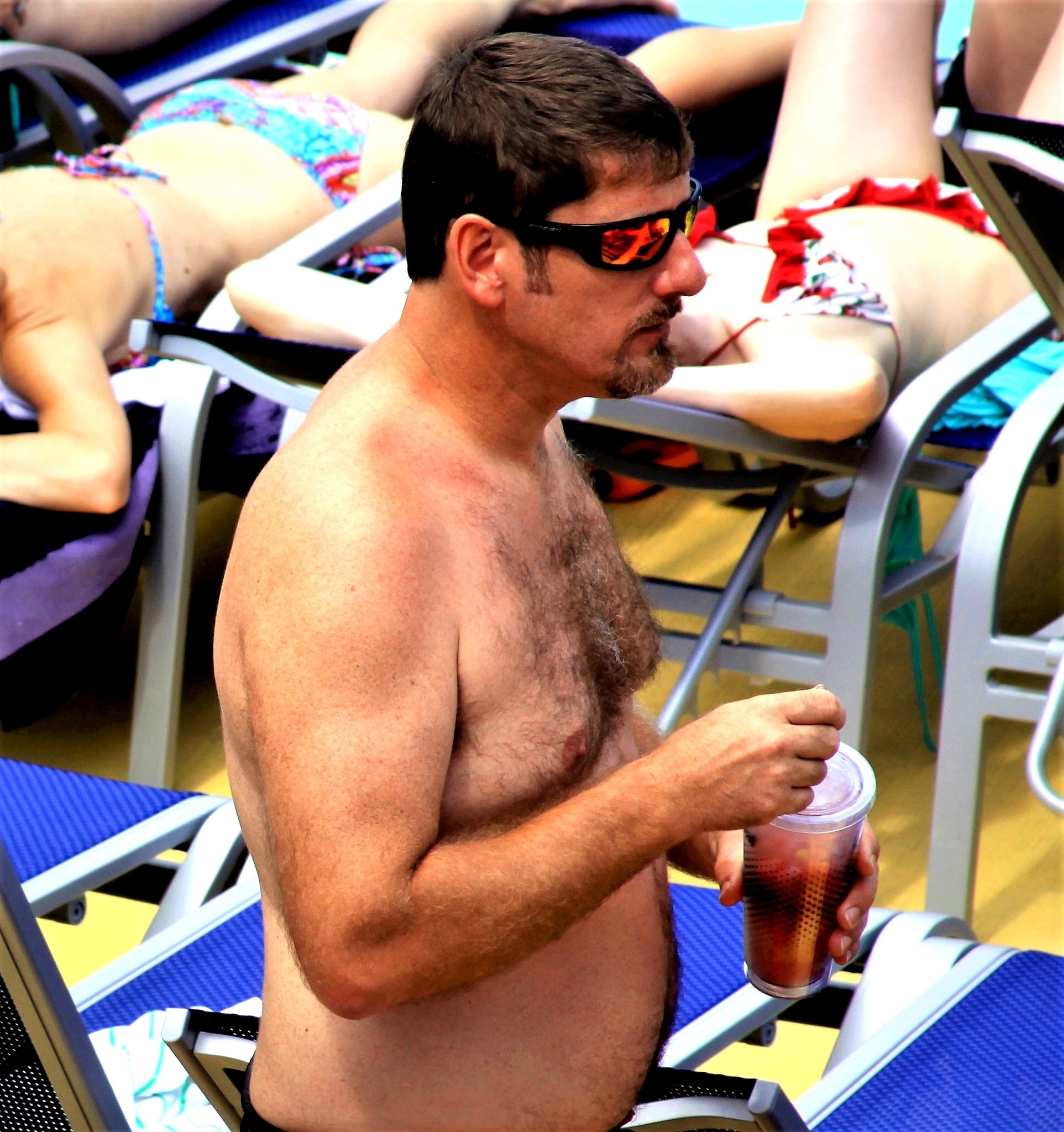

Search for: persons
xmin=213 ymin=35 xmax=881 ymax=1132
xmin=1 ymin=1 xmax=1063 ymax=520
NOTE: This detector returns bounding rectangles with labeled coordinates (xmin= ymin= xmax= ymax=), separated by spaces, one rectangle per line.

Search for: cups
xmin=743 ymin=741 xmax=877 ymax=999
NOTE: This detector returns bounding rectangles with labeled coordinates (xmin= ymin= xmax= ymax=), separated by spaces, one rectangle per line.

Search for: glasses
xmin=448 ymin=173 xmax=703 ymax=270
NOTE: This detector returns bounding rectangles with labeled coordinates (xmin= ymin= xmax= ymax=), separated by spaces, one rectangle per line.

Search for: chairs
xmin=1 ymin=3 xmax=1063 ymax=1132
